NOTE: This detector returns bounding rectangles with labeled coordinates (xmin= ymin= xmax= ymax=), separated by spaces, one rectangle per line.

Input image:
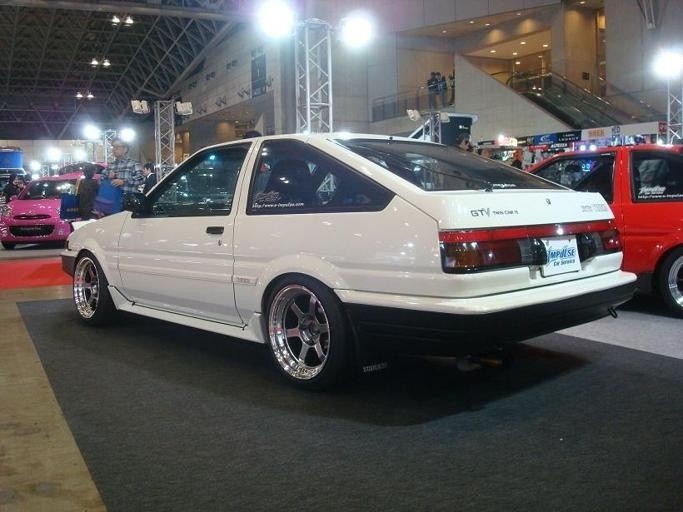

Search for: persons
xmin=244 ymin=130 xmax=261 ymax=138
xmin=637 ymin=160 xmax=670 ymax=192
xmin=142 ymin=163 xmax=157 ymax=194
xmin=109 ymin=138 xmax=146 ymax=216
xmin=25 ymin=180 xmax=30 ymax=196
xmin=426 ymin=64 xmax=457 ymax=107
xmin=511 ymin=147 xmax=525 ymax=169
xmin=479 ymin=146 xmax=492 ymax=161
xmin=6 ymin=172 xmax=23 ymax=202
xmin=77 ymin=164 xmax=100 ymax=219
xmin=565 ymin=166 xmax=584 ymax=184
xmin=461 ymin=137 xmax=472 ymax=153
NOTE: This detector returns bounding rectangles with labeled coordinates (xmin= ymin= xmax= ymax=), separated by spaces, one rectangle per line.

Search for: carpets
xmin=16 ymin=296 xmax=681 ymax=511
xmin=0 ymin=258 xmax=73 ymax=289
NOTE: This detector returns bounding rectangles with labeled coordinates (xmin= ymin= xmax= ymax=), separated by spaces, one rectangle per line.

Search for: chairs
xmin=604 ymin=164 xmax=641 ymax=200
xmin=261 ymin=159 xmax=422 ymax=206
